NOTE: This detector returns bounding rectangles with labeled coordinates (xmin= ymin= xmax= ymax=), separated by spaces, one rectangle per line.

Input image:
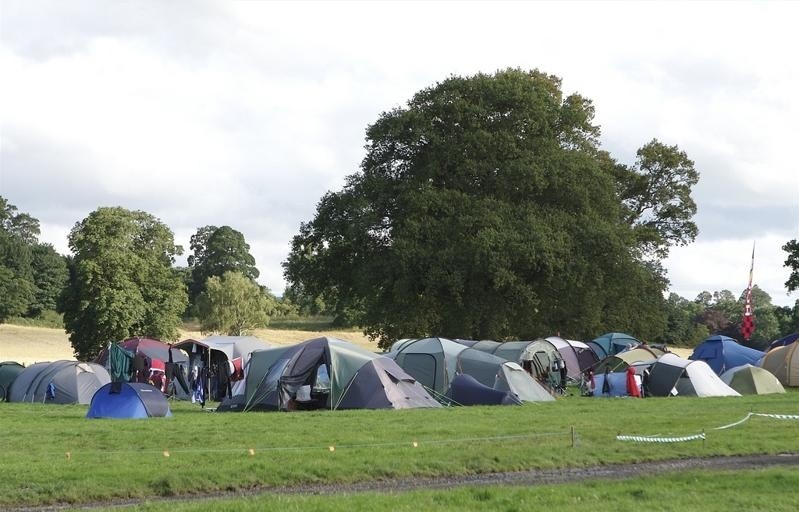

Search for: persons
xmin=131 ymin=370 xmax=166 ymax=392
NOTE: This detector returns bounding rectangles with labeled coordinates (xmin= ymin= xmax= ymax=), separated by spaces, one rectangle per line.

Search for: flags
xmin=740 ymin=248 xmax=759 ymax=343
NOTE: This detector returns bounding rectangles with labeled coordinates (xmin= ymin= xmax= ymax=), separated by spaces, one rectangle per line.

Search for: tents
xmin=214 ymin=334 xmax=556 ymax=412
xmin=720 ymin=363 xmax=787 ymax=395
xmin=542 ymin=333 xmax=743 ymax=398
xmin=688 ymin=334 xmax=767 ymax=379
xmin=165 ymin=335 xmax=277 ymax=401
xmin=754 ymin=332 xmax=799 ymax=386
xmin=104 ymin=341 xmax=134 ymax=382
xmin=0 ymin=360 xmax=112 ymax=405
xmin=86 ymin=383 xmax=173 ymax=418
xmin=92 ymin=336 xmax=190 ymax=371
